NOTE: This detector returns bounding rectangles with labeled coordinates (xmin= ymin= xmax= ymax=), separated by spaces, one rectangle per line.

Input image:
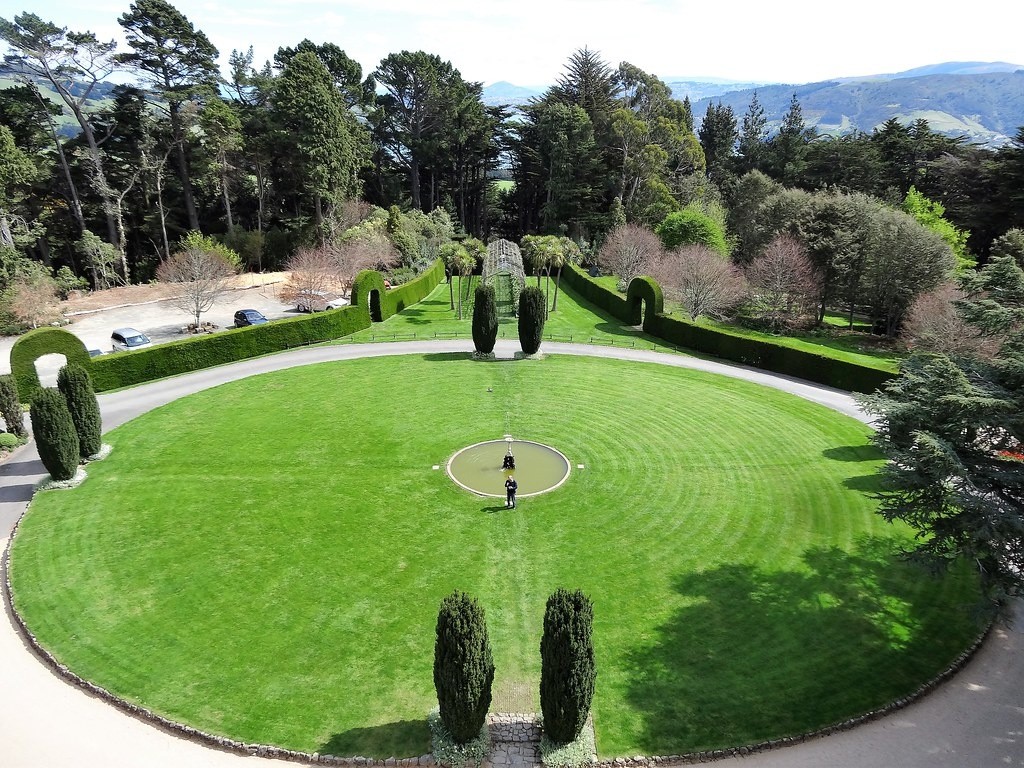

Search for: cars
xmin=86 ymin=347 xmax=108 ymax=356
xmin=234 ymin=308 xmax=270 ymax=328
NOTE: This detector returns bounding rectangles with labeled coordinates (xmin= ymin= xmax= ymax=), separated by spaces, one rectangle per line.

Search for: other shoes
xmin=507 ymin=506 xmax=511 ymax=509
xmin=513 ymin=507 xmax=516 ymax=510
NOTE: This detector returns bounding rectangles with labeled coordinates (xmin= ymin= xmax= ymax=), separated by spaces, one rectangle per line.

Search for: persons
xmin=504 ymin=475 xmax=518 ymax=510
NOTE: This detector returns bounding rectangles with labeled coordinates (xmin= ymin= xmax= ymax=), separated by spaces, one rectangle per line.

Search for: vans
xmin=112 ymin=327 xmax=153 ymax=351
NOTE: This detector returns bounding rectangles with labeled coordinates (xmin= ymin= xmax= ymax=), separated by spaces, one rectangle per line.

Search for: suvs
xmin=292 ymin=288 xmax=348 ymax=313
